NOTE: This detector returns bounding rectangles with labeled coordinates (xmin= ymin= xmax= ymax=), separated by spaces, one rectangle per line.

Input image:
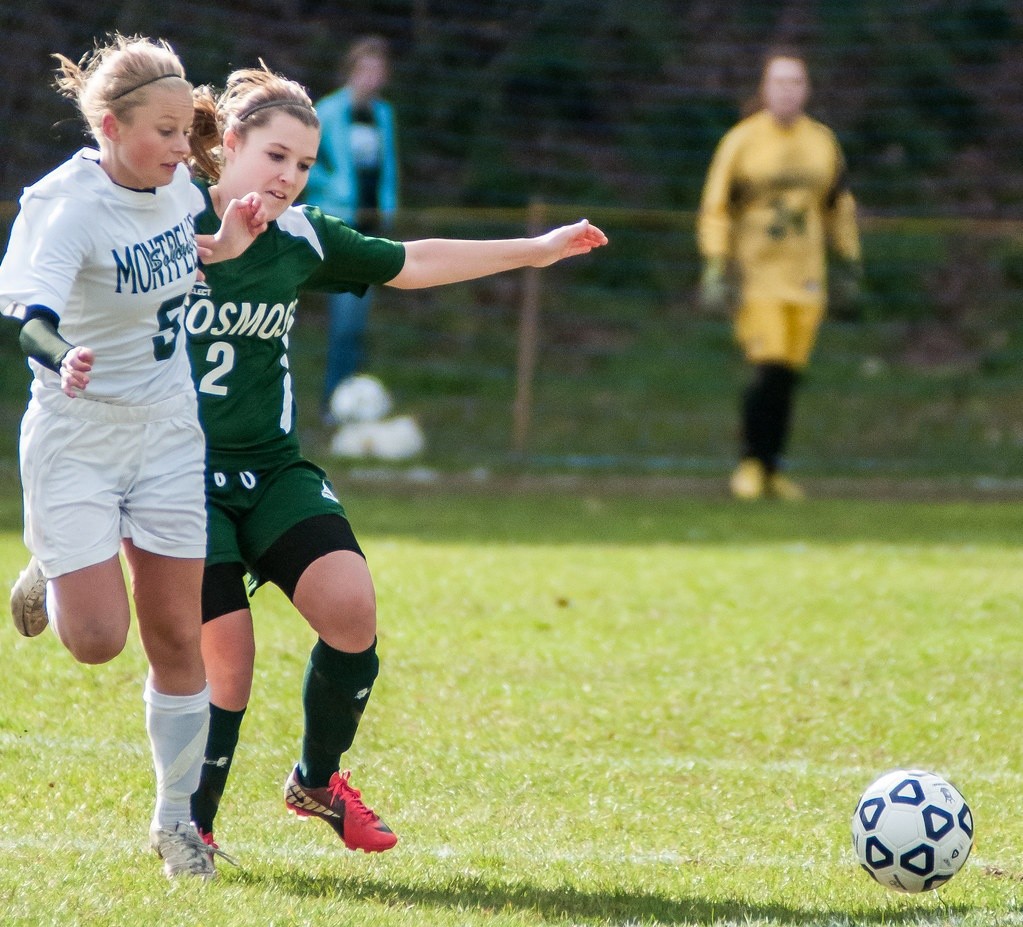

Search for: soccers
xmin=850 ymin=767 xmax=975 ymax=895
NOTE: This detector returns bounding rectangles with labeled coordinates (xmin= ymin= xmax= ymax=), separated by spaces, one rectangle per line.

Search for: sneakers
xmin=149 ymin=818 xmax=214 ymax=888
xmin=9 ymin=554 xmax=48 ymax=637
xmin=285 ymin=764 xmax=398 ymax=853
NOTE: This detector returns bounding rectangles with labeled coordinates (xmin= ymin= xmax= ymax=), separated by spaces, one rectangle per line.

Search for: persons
xmin=695 ymin=44 xmax=863 ymax=508
xmin=0 ymin=31 xmax=214 ymax=881
xmin=301 ymin=35 xmax=397 ymax=424
xmin=180 ymin=56 xmax=607 ymax=854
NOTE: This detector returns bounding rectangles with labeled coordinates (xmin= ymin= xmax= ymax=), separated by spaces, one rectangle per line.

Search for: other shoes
xmin=771 ymin=475 xmax=803 ymax=502
xmin=730 ymin=459 xmax=764 ymax=502
